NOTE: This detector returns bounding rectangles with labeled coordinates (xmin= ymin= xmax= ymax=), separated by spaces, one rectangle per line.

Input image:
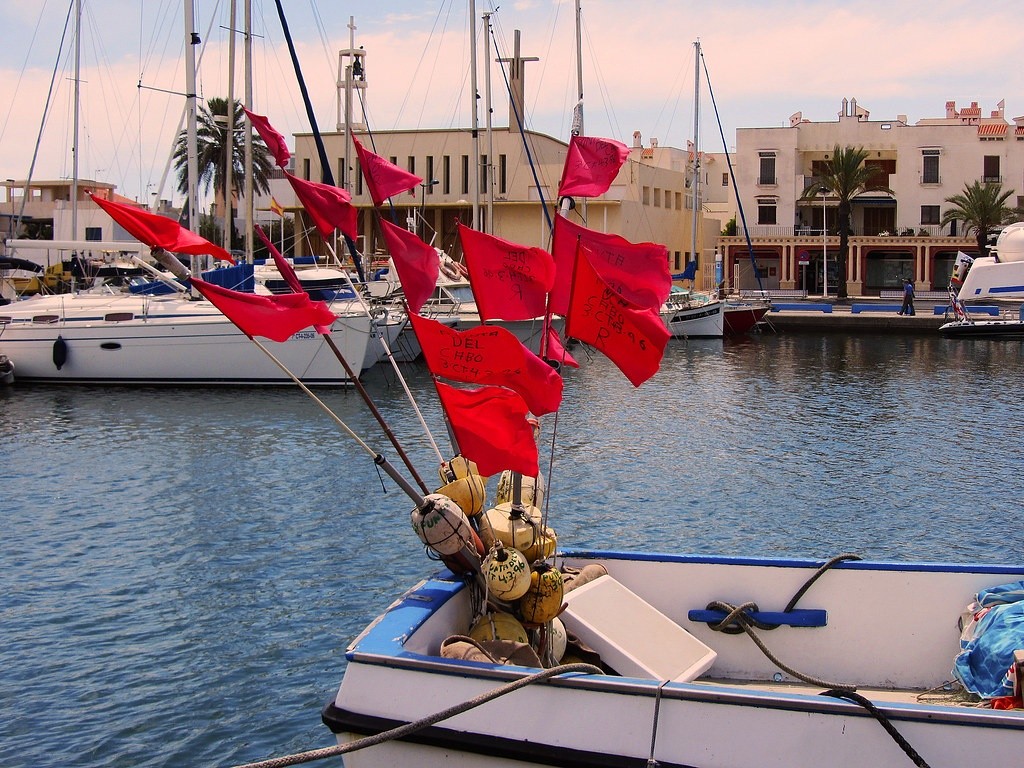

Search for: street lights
xmin=818 ymin=187 xmax=831 ymax=297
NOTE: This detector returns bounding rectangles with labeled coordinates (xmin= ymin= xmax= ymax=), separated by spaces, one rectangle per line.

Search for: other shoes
xmin=897 ymin=312 xmax=902 ymax=315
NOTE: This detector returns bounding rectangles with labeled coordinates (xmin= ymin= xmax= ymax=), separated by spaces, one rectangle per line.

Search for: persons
xmin=897 ymin=280 xmax=915 ymax=316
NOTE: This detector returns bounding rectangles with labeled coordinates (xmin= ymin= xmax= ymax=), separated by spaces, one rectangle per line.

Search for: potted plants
xmin=900 ymin=228 xmax=914 ymax=236
xmin=918 ymin=227 xmax=930 ymax=236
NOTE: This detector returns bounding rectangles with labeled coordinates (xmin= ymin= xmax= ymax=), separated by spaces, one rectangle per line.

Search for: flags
xmin=381 ymin=218 xmax=440 ymax=314
xmin=549 ymin=213 xmax=671 ymax=317
xmin=561 ymin=137 xmax=632 ymax=196
xmin=568 ymin=245 xmax=671 ymax=388
xmin=290 ymin=172 xmax=357 ymax=241
xmin=91 ymin=194 xmax=237 ymax=265
xmin=258 ymin=227 xmax=331 ymax=335
xmin=407 ymin=311 xmax=563 ymax=416
xmin=192 ymin=277 xmax=338 ymax=343
xmin=271 ymin=196 xmax=284 ymax=217
xmin=244 ymin=108 xmax=290 ymax=166
xmin=458 ymin=222 xmax=556 ymax=320
xmin=353 ymin=135 xmax=423 ymax=203
xmin=436 ymin=381 xmax=538 ymax=478
xmin=548 ymin=326 xmax=579 ymax=369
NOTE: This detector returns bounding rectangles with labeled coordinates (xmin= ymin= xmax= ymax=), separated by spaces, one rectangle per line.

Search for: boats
xmin=937 ymin=222 xmax=1024 ymax=334
xmin=319 ymin=545 xmax=1024 ymax=768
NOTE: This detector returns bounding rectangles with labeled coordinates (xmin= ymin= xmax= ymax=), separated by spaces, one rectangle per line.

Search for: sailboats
xmin=0 ymin=0 xmax=770 ymax=389
